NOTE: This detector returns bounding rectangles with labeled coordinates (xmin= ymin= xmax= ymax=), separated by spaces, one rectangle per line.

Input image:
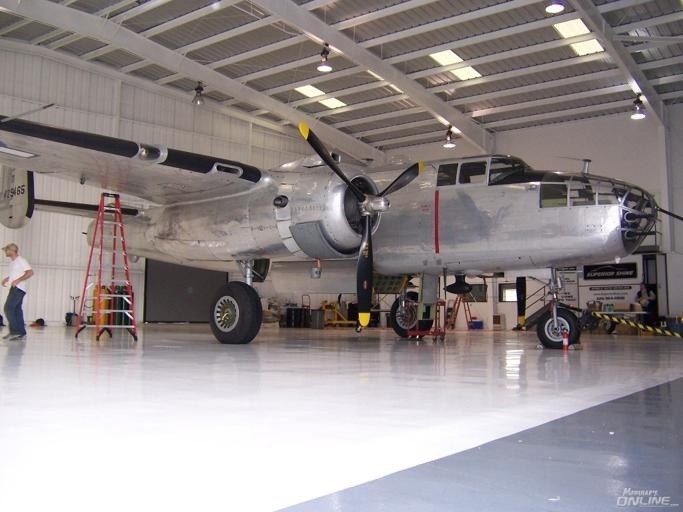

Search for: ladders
xmin=75 ymin=193 xmax=136 ymax=340
xmin=447 ymin=294 xmax=472 ymax=330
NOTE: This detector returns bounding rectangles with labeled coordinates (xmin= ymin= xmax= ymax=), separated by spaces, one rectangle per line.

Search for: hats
xmin=2 ymin=243 xmax=18 ymax=252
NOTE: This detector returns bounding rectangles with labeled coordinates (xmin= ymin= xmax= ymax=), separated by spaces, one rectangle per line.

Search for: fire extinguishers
xmin=97 ymin=285 xmax=133 ymax=327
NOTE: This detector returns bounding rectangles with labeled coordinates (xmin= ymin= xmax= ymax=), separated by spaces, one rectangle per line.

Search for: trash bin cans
xmin=311 ymin=309 xmax=322 ymax=328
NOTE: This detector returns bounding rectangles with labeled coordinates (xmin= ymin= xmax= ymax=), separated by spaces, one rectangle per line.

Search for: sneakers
xmin=2 ymin=331 xmax=26 ymax=341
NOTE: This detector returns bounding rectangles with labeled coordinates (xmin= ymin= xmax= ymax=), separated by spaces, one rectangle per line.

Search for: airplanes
xmin=0 ymin=113 xmax=660 ymax=350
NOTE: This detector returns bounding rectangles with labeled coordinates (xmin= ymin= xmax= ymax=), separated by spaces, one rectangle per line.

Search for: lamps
xmin=192 ymin=81 xmax=205 ymax=106
xmin=316 ymin=43 xmax=334 ymax=72
xmin=630 ymin=93 xmax=646 ymax=120
xmin=443 ymin=125 xmax=456 ymax=148
xmin=545 ymin=0 xmax=565 ymax=13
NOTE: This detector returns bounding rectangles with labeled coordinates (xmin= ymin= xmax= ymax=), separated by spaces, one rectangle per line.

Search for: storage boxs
xmin=616 ymin=324 xmax=636 ymax=336
xmin=468 ymin=320 xmax=483 ymax=329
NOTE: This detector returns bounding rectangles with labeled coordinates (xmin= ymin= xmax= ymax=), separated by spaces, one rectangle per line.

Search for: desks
xmin=590 ymin=311 xmax=648 ymax=335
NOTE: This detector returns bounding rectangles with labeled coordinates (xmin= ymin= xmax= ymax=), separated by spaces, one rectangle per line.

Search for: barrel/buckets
xmin=312 ymin=310 xmax=324 ymax=329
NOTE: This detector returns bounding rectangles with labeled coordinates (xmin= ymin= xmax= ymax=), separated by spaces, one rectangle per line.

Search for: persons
xmin=0 ymin=243 xmax=34 ymax=340
xmin=632 ymin=281 xmax=655 ymax=324
